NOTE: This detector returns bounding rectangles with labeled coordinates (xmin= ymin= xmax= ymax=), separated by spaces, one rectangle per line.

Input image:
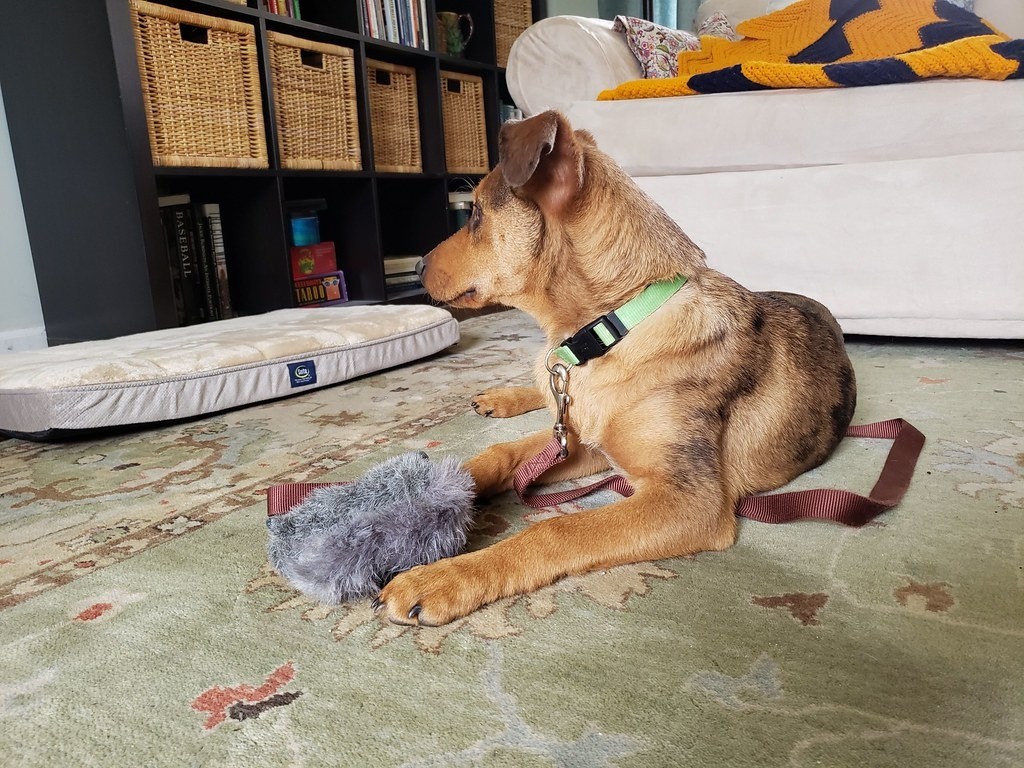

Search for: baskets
xmin=440 ymin=70 xmax=491 ymax=175
xmin=132 ymin=1 xmax=270 ymax=168
xmin=367 ymin=57 xmax=424 ymax=174
xmin=267 ymin=30 xmax=363 ymax=171
xmin=494 ymin=0 xmax=533 ymax=69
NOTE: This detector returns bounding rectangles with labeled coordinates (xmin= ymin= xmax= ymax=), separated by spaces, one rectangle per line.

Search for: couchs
xmin=505 ymin=0 xmax=1024 ymax=343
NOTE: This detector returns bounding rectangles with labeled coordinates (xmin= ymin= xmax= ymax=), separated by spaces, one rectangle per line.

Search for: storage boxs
xmin=288 ymin=218 xmax=348 ymax=307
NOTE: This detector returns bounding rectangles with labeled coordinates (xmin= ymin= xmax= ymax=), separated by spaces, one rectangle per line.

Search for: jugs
xmin=437 ymin=12 xmax=474 ymax=57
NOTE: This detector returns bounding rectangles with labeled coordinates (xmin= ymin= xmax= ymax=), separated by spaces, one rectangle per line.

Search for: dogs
xmin=369 ymin=107 xmax=860 ymax=630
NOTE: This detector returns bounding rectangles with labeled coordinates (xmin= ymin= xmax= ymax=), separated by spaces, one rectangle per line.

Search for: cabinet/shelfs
xmin=0 ymin=0 xmax=548 ymax=346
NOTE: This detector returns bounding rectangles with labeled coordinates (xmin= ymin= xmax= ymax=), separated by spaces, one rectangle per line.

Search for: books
xmin=500 ymin=101 xmax=524 ymax=122
xmin=362 ymin=0 xmax=430 ymax=51
xmin=157 ymin=194 xmax=232 ymax=324
xmin=267 ymin=0 xmax=301 ymax=20
xmin=383 ymin=256 xmax=425 ymax=292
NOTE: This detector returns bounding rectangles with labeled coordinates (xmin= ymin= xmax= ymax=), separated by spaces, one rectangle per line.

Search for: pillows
xmin=611 ymin=13 xmax=736 ymax=79
